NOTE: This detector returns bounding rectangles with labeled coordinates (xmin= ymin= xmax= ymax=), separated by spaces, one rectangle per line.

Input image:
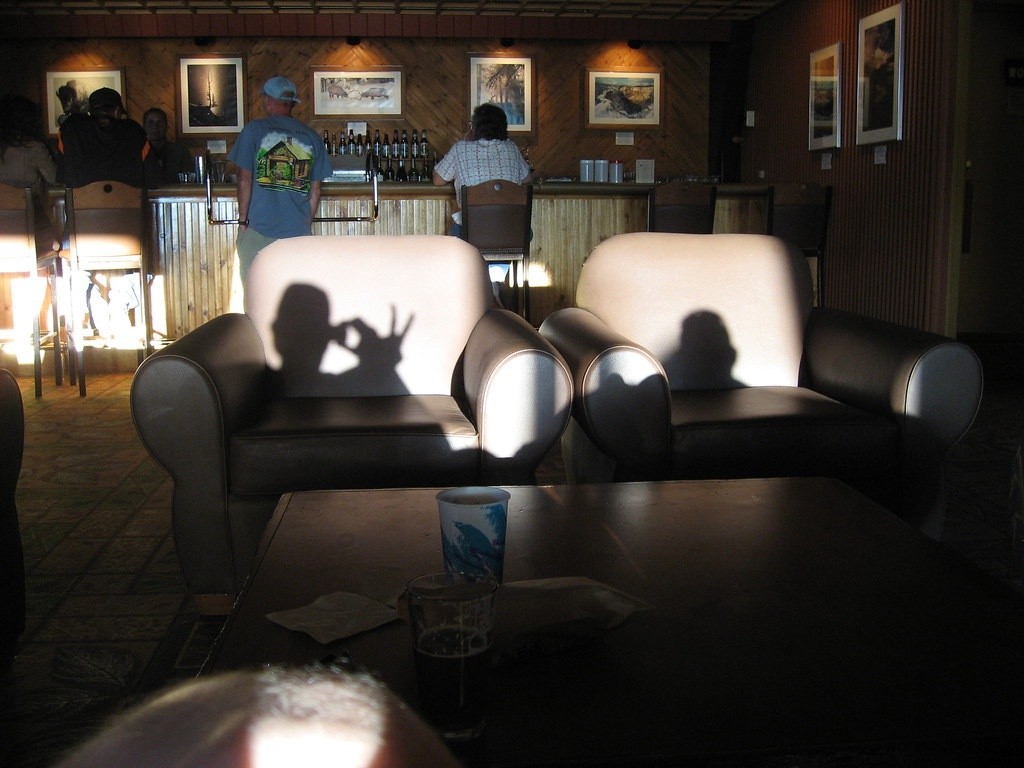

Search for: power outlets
xmin=821 ymin=152 xmax=832 ymax=170
xmin=874 ymin=146 xmax=887 ymax=164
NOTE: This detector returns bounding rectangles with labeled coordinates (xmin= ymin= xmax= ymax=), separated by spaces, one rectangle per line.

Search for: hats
xmin=257 ymin=75 xmax=301 ymax=105
xmin=88 ymin=88 xmax=128 ymax=117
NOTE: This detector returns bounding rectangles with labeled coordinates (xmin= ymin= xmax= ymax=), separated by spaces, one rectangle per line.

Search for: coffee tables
xmin=196 ymin=476 xmax=1024 ymax=768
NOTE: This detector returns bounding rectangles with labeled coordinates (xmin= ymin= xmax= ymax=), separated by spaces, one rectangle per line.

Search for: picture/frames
xmin=464 ymin=52 xmax=538 ymax=138
xmin=583 ymin=66 xmax=666 ymax=131
xmin=43 ymin=65 xmax=126 ymax=141
xmin=308 ymin=64 xmax=407 ymax=122
xmin=174 ymin=51 xmax=249 ymax=140
xmin=856 ymin=0 xmax=903 ymax=145
xmin=808 ymin=41 xmax=841 ymax=151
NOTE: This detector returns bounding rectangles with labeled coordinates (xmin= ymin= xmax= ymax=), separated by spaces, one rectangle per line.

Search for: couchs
xmin=537 ymin=232 xmax=985 ymax=546
xmin=130 ymin=234 xmax=576 ymax=623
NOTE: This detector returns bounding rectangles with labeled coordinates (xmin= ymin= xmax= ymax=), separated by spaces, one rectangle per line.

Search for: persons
xmin=0 ymin=94 xmax=57 ymax=343
xmin=227 ymin=76 xmax=333 ymax=292
xmin=60 ymin=88 xmax=155 ymax=336
xmin=433 ymin=103 xmax=532 ymax=308
xmin=143 ymin=108 xmax=188 ymax=174
xmin=55 ymin=667 xmax=463 ymax=768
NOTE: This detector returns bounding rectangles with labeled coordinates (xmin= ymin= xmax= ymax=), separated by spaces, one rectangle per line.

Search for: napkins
xmin=267 ymin=591 xmax=399 ymax=644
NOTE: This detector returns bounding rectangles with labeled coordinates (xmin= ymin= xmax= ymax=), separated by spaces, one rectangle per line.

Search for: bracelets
xmin=238 ymin=219 xmax=246 ymax=225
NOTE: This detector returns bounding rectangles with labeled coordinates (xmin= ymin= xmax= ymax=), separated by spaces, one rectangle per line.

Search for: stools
xmin=647 ymin=180 xmax=718 ymax=234
xmin=766 ymin=183 xmax=833 ymax=309
xmin=0 ymin=178 xmax=159 ymax=399
xmin=462 ymin=179 xmax=533 ymax=326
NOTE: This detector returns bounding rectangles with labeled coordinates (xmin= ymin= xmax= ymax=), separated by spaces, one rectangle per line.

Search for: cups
xmin=178 ymin=173 xmax=196 ymax=183
xmin=404 ymin=571 xmax=498 ymax=739
xmin=231 ymin=174 xmax=239 ymax=183
xmin=435 ymin=486 xmax=511 ymax=585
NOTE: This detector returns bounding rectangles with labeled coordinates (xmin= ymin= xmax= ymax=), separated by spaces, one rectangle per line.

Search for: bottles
xmin=420 ymin=130 xmax=428 ymax=158
xmin=610 ymin=160 xmax=623 ymax=183
xmin=595 ymin=160 xmax=609 ymax=183
xmin=195 ymin=156 xmax=206 ymax=183
xmin=385 ymin=159 xmax=395 ymax=182
xmin=382 ymin=134 xmax=390 ymax=158
xmin=422 ymin=166 xmax=431 ymax=182
xmin=392 ymin=130 xmax=400 ymax=158
xmin=409 ymin=158 xmax=419 ymax=182
xmin=580 ymin=159 xmax=594 ymax=183
xmin=364 ymin=130 xmax=372 ymax=158
xmin=412 ymin=130 xmax=419 ymax=158
xmin=401 ymin=130 xmax=408 ymax=158
xmin=213 ymin=161 xmax=225 ymax=184
xmin=322 ymin=130 xmax=338 ymax=158
xmin=339 ymin=130 xmax=347 ymax=156
xmin=366 ymin=158 xmax=385 ymax=182
xmin=396 ymin=160 xmax=407 ymax=181
xmin=347 ymin=130 xmax=356 ymax=156
xmin=373 ymin=130 xmax=382 ymax=157
xmin=356 ymin=130 xmax=364 ymax=157
